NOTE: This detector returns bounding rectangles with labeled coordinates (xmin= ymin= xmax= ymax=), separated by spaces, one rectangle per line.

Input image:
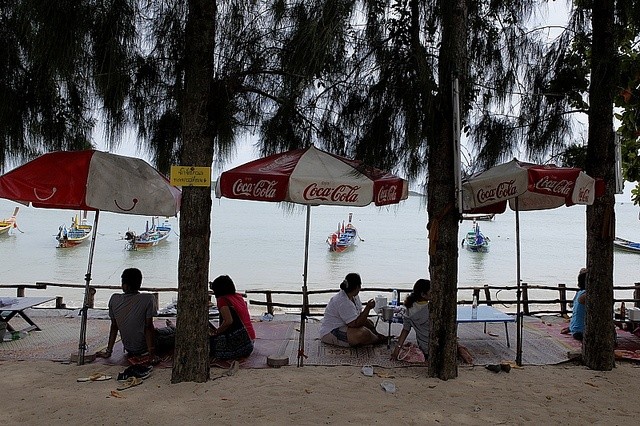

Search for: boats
xmin=326 ymin=212 xmax=364 ymax=253
xmin=0 ymin=205 xmax=23 ymax=237
xmin=461 ymin=222 xmax=490 ymax=254
xmin=55 ymin=208 xmax=92 ymax=246
xmin=614 ymin=234 xmax=640 ymax=253
xmin=125 ymin=218 xmax=172 ymax=252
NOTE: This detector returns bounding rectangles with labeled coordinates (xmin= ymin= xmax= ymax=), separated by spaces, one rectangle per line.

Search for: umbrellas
xmin=215 ymin=142 xmax=408 ymax=368
xmin=461 ymin=156 xmax=604 ymax=366
xmin=0 ymin=149 xmax=182 ymax=366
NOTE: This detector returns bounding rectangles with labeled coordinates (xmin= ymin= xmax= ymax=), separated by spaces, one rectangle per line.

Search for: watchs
xmin=395 ymin=343 xmax=402 ymax=348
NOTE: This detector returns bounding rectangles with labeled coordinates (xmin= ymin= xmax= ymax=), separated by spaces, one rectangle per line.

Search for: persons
xmin=390 ymin=279 xmax=477 ymax=364
xmin=95 ymin=268 xmax=176 ymax=365
xmin=319 ymin=273 xmax=396 ymax=348
xmin=569 ymin=267 xmax=586 ymax=341
xmin=208 ymin=275 xmax=256 ymax=361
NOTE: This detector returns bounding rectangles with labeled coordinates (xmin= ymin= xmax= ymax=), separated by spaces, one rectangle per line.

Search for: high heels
xmin=500 ymin=363 xmax=511 ymax=373
xmin=485 ymin=364 xmax=501 ymax=373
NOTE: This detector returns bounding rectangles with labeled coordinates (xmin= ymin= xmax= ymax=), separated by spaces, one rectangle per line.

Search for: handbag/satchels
xmin=214 ymin=306 xmax=254 ymax=358
xmin=397 ymin=342 xmax=425 ymax=362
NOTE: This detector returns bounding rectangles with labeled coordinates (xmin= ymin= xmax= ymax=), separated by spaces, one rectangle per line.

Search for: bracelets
xmin=107 ymin=347 xmax=114 ymax=352
xmin=106 ymin=350 xmax=112 ymax=353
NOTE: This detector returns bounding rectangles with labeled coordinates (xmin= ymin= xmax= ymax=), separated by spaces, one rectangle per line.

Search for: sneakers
xmin=117 ymin=364 xmax=153 ymax=381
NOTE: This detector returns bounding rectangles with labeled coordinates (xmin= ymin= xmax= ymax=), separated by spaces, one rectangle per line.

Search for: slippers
xmin=380 ymin=380 xmax=396 ymax=392
xmin=77 ymin=372 xmax=112 ymax=381
xmin=361 ymin=364 xmax=373 ymax=376
xmin=117 ymin=377 xmax=143 ymax=389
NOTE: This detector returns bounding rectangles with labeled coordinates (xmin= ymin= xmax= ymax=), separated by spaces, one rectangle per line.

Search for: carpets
xmin=284 ymin=321 xmax=580 ymax=368
xmin=522 ymin=322 xmax=640 ymax=362
xmin=88 ymin=317 xmax=298 ymax=369
xmin=1 ymin=317 xmax=122 ymax=361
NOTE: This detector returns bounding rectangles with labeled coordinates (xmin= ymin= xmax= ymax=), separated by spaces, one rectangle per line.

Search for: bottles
xmin=621 ymin=302 xmax=625 ymax=319
xmin=471 ymin=296 xmax=478 ymax=319
xmin=3 ymin=331 xmax=33 ymax=340
xmin=392 ymin=289 xmax=398 ymax=306
